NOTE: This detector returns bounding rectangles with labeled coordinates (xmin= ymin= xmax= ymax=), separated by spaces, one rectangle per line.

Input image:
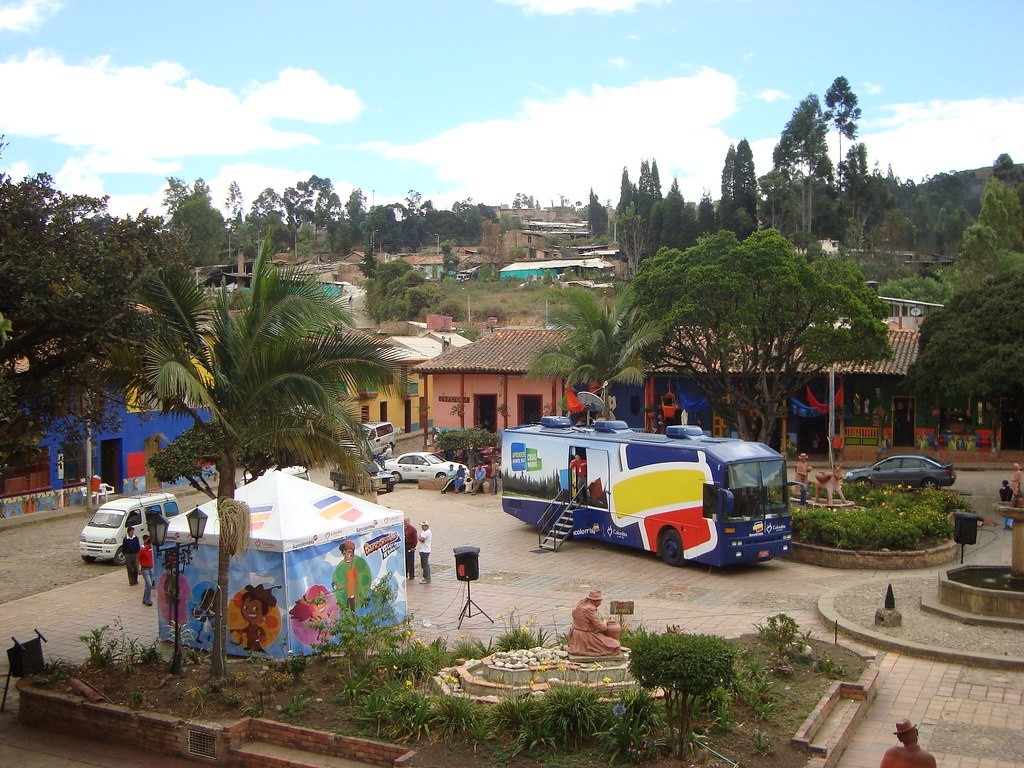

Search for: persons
xmin=569 ymin=590 xmax=621 ymax=656
xmin=570 ymin=454 xmax=587 ymax=505
xmin=404 ymin=517 xmax=417 ymax=580
xmin=136 ymin=538 xmax=153 ymax=606
xmin=441 ymin=464 xmax=458 ymax=494
xmin=795 ymin=453 xmax=812 ymax=494
xmin=999 ymin=480 xmax=1015 ymax=530
xmin=1012 ymin=462 xmax=1023 ymax=495
xmin=382 ymin=444 xmax=392 ymax=460
xmin=464 ymin=464 xmax=486 ymax=494
xmin=453 ymin=465 xmax=465 ymax=493
xmin=418 ymin=521 xmax=432 ymax=583
xmin=123 ymin=527 xmax=141 ymax=586
xmin=880 ymin=719 xmax=937 ymax=768
xmin=491 ymin=457 xmax=499 ymax=495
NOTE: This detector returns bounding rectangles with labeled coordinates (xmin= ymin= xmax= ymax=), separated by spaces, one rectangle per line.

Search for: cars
xmin=329 ymin=461 xmax=396 ymax=495
xmin=841 ymin=455 xmax=957 ymax=490
xmin=385 ymin=452 xmax=470 ymax=483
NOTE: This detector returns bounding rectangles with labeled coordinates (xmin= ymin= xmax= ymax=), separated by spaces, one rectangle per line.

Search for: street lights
xmin=435 ymin=234 xmax=439 ymax=254
xmin=147 ymin=508 xmax=209 ymax=674
xmin=373 ymin=230 xmax=379 ymax=255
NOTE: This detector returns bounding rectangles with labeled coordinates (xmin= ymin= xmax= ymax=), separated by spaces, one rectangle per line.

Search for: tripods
xmin=457 ymin=581 xmax=494 ymax=629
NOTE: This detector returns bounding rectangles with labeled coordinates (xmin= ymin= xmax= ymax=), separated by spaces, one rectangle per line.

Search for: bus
xmin=498 ymin=416 xmax=808 ymax=568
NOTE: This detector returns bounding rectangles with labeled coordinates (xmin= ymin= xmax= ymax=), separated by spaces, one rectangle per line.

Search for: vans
xmin=337 ymin=422 xmax=397 ymax=462
xmin=78 ymin=492 xmax=180 ymax=567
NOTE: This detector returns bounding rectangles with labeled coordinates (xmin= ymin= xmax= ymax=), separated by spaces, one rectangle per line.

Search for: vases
xmin=605 ymin=622 xmax=621 ymax=640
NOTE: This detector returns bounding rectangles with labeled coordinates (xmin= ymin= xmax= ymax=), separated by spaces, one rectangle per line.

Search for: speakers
xmin=453 ymin=546 xmax=480 ymax=581
xmin=954 ymin=513 xmax=977 ymax=545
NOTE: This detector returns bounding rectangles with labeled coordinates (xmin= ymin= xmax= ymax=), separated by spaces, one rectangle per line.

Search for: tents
xmin=154 ymin=468 xmax=408 ymax=661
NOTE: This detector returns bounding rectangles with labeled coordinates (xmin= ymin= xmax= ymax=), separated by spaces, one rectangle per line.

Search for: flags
xmin=566 ymin=389 xmax=585 ymax=414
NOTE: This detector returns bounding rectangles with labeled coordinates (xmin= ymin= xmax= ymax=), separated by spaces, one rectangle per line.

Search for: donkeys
xmin=815 ymin=464 xmax=846 ymax=505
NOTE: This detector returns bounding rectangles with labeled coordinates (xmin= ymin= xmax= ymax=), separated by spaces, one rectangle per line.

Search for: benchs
xmin=418 ymin=478 xmax=492 ymax=491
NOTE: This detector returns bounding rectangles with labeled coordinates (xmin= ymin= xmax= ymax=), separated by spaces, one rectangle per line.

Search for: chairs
xmin=81 ymin=483 xmax=114 ymax=505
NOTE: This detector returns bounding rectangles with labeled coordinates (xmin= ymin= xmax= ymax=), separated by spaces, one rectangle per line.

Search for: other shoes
xmin=455 ymin=490 xmax=459 ymax=493
xmin=130 ymin=582 xmax=140 ymax=586
xmin=441 ymin=490 xmax=446 ymax=494
xmin=409 ymin=577 xmax=414 ymax=580
xmin=470 ymin=491 xmax=477 ymax=495
xmin=143 ymin=599 xmax=153 ymax=607
xmin=419 ymin=578 xmax=432 ymax=584
xmin=491 ymin=492 xmax=497 ymax=495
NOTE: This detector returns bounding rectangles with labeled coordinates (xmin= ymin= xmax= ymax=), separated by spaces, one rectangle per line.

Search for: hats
xmin=419 ymin=521 xmax=429 ymax=526
